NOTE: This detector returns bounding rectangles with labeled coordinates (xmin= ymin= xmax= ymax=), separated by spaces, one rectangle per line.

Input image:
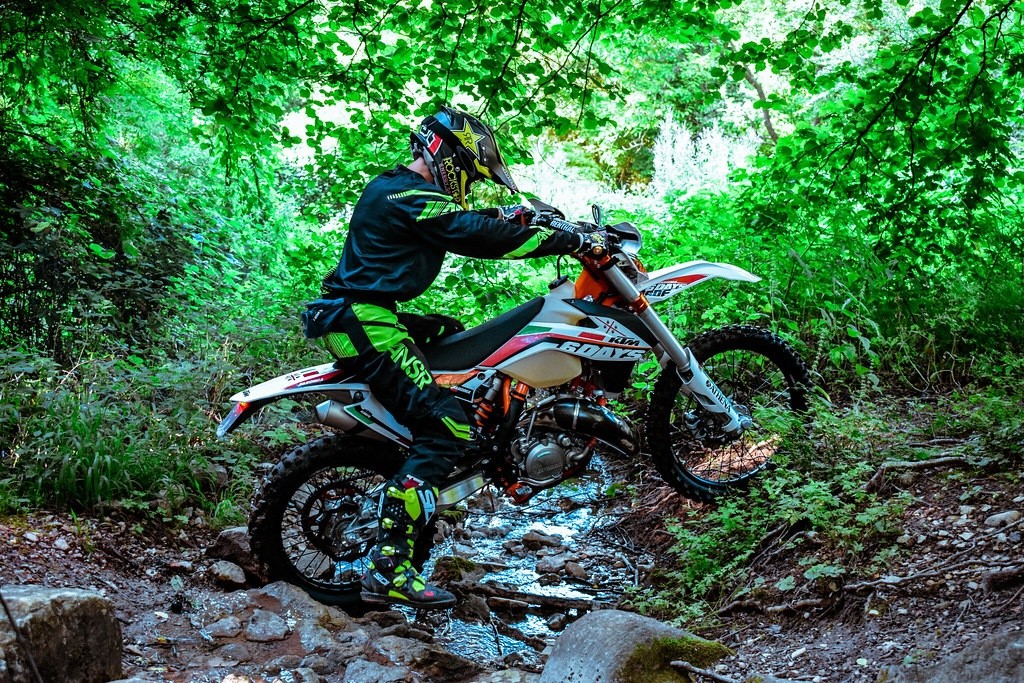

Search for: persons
xmin=321 ymin=105 xmax=607 ymax=609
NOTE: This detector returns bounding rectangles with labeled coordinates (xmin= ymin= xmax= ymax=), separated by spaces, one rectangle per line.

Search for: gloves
xmin=501 ymin=204 xmax=536 ymax=225
xmin=572 ymin=225 xmax=621 ymax=258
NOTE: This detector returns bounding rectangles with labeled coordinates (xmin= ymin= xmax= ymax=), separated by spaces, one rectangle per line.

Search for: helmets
xmin=410 ymin=104 xmax=520 ymax=213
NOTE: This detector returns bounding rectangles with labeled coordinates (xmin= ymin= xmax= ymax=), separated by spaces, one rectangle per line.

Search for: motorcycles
xmin=215 ymin=209 xmax=813 ymax=617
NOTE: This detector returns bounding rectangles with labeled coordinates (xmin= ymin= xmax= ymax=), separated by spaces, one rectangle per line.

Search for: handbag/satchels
xmin=302 ymin=295 xmax=397 ymax=337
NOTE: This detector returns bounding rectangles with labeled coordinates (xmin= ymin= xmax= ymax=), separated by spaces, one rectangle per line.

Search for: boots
xmin=335 ymin=484 xmax=387 ymax=581
xmin=360 ymin=473 xmax=457 ymax=609
